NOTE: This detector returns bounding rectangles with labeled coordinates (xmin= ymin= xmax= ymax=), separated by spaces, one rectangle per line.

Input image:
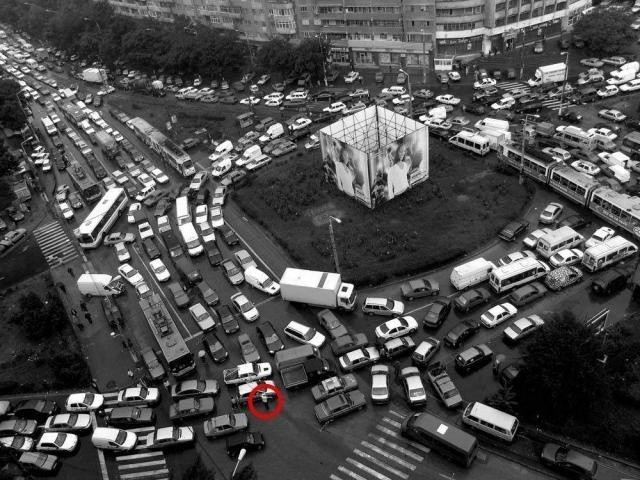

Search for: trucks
xmin=281 ymin=268 xmax=358 ymax=315
xmin=449 ymin=258 xmax=495 ymax=291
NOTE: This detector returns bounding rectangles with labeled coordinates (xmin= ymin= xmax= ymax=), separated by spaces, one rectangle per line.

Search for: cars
xmin=498 ymin=249 xmax=538 ymax=266
xmin=451 ymin=288 xmax=491 ymax=308
xmin=522 ymin=228 xmax=553 ymax=247
xmin=584 ymin=227 xmax=616 ymax=251
xmin=499 ymin=217 xmax=529 ymax=241
xmin=549 ymin=249 xmax=585 ymax=266
xmin=278 ymin=302 xmax=545 ymax=424
xmin=279 ymin=1 xmax=640 ymax=196
xmin=538 ymin=201 xmax=563 ymax=226
xmin=0 ymin=24 xmax=278 ymax=473
xmin=557 ymin=214 xmax=591 ymax=229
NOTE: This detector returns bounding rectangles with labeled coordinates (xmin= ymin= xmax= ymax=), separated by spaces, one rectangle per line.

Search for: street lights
xmin=44 ymin=9 xmax=57 ymax=15
xmin=24 ymin=3 xmax=37 ymax=9
xmin=231 ymin=448 xmax=247 ymax=479
xmin=84 ymin=18 xmax=101 ymax=32
xmin=328 ymin=215 xmax=342 ymax=281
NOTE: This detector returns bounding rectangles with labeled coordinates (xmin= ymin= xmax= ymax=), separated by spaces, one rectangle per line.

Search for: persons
xmin=56 ymin=281 xmax=66 ymax=294
xmin=333 ymin=148 xmax=355 ymax=197
xmin=104 ymin=414 xmax=111 ymax=426
xmin=80 ymin=300 xmax=88 ymax=312
xmin=261 ymin=392 xmax=270 ymax=410
xmin=73 ymin=317 xmax=86 ymax=331
xmin=71 ymin=308 xmax=77 ymax=316
xmin=391 ymin=359 xmax=401 ymax=377
xmin=83 ymin=310 xmax=94 ymax=324
xmin=92 ymin=379 xmax=100 ymax=392
xmin=67 ymin=266 xmax=74 ymax=276
xmin=230 ymin=396 xmax=239 ymax=409
xmin=385 ymin=143 xmax=411 ymax=199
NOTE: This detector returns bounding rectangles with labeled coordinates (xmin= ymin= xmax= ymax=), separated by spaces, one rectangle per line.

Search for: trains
xmin=494 ymin=139 xmax=640 ymax=240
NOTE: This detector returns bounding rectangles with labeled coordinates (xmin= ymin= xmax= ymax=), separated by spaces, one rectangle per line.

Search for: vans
xmin=462 ymin=401 xmax=520 ymax=443
xmin=581 ymin=235 xmax=639 ymax=271
xmin=402 ymin=412 xmax=479 ymax=469
xmin=535 ymin=226 xmax=586 ymax=258
xmin=489 ymin=258 xmax=550 ymax=294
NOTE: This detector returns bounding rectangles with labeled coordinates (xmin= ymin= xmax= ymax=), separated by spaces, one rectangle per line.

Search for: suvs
xmin=511 ymin=281 xmax=547 ymax=307
xmin=541 ymin=441 xmax=597 ymax=478
xmin=400 ymin=277 xmax=441 ymax=300
xmin=361 ymin=298 xmax=405 ymax=318
xmin=544 ymin=266 xmax=583 ymax=292
xmin=592 ymin=266 xmax=631 ymax=296
